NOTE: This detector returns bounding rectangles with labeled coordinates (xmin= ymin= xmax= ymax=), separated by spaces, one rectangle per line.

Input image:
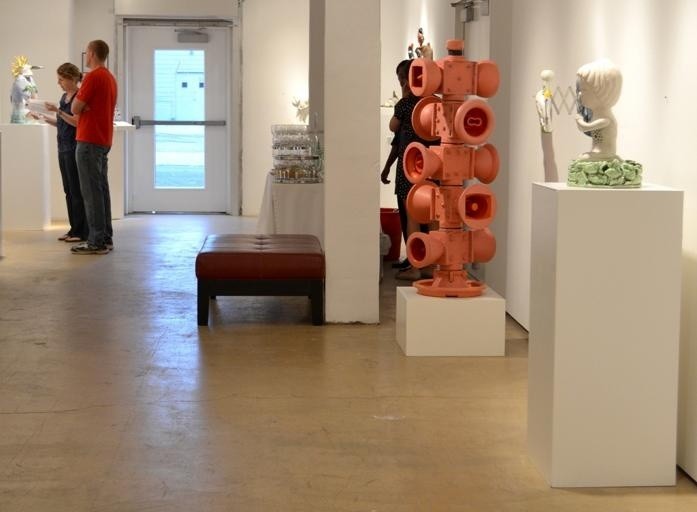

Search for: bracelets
xmin=58 ymin=108 xmax=64 ymax=116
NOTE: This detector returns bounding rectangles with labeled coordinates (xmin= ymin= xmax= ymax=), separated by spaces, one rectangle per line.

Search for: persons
xmin=381 ymin=59 xmax=410 ymax=268
xmin=30 ymin=63 xmax=89 ymax=242
xmin=391 ymin=61 xmax=442 ymax=280
xmin=70 ymin=40 xmax=118 ymax=254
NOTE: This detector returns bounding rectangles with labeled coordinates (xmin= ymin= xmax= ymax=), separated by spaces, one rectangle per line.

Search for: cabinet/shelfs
xmin=258 ymin=174 xmax=323 ymax=235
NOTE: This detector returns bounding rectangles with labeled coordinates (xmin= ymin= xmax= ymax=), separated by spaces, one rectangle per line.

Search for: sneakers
xmin=58 ymin=233 xmax=113 ymax=254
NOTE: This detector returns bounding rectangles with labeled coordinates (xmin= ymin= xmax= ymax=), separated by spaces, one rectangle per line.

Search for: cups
xmin=272 ymin=157 xmax=321 ymax=184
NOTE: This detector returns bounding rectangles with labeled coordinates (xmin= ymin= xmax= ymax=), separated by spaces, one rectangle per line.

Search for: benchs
xmin=196 ymin=235 xmax=324 ymax=326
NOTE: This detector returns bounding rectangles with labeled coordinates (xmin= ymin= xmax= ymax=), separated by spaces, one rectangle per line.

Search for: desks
xmin=0 ymin=124 xmax=136 ymax=231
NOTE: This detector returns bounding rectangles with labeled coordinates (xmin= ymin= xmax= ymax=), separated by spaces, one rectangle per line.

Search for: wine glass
xmin=272 ymin=124 xmax=319 ymax=155
